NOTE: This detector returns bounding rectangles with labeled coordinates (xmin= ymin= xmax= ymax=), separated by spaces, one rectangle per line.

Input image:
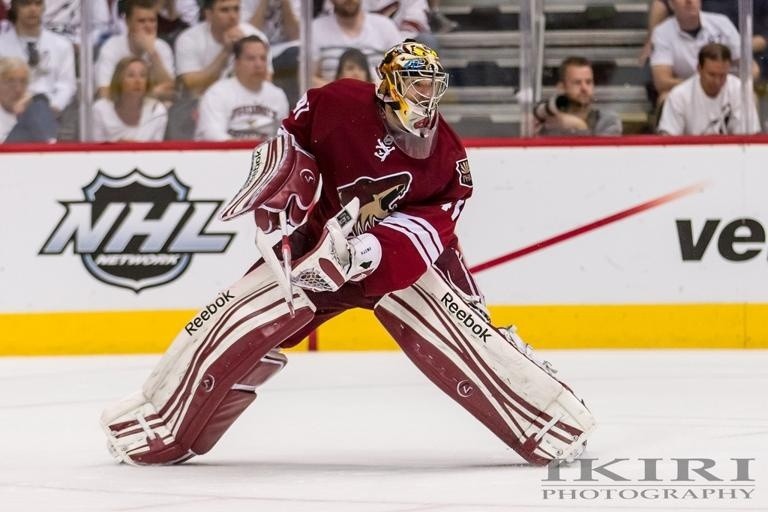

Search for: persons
xmin=1 ymin=55 xmax=61 ymax=145
xmin=174 ymin=2 xmax=275 ymax=130
xmin=195 ymin=37 xmax=291 ymax=143
xmin=640 ymin=0 xmax=768 ymax=103
xmin=0 ymin=0 xmax=76 ymax=115
xmin=92 ymin=1 xmax=176 ymax=109
xmin=532 ymin=61 xmax=622 ymax=138
xmin=426 ymin=1 xmax=459 ymax=35
xmin=88 ymin=57 xmax=169 ymax=145
xmin=331 ymin=46 xmax=377 ymax=85
xmin=649 ymin=0 xmax=763 ymax=124
xmin=239 ymin=0 xmax=303 ymax=77
xmin=101 ymin=40 xmax=594 ymax=468
xmin=660 ymin=45 xmax=763 ymax=137
xmin=299 ymin=0 xmax=401 ymax=90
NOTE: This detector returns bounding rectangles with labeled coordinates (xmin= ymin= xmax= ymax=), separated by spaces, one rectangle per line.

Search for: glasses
xmin=27 ymin=41 xmax=39 ymax=67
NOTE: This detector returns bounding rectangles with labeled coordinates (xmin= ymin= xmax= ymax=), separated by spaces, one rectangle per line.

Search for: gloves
xmin=218 ymin=134 xmax=323 ymax=234
xmin=289 ymin=196 xmax=382 ymax=292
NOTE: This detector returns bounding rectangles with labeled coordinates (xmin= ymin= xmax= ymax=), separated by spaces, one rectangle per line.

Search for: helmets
xmin=373 ymin=42 xmax=449 ymax=159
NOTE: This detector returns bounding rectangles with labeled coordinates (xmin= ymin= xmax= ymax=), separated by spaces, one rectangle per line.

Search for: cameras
xmin=534 ymin=95 xmax=573 ymax=120
